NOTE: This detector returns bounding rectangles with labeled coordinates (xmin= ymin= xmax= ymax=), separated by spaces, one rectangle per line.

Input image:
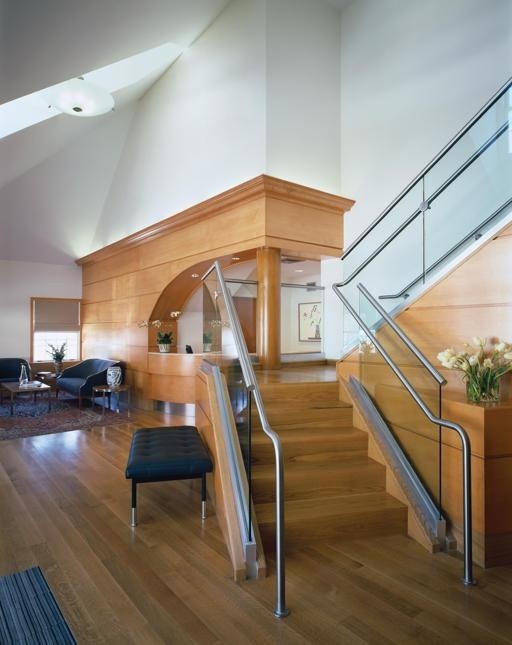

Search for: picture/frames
xmin=297 ymin=303 xmax=323 ymax=343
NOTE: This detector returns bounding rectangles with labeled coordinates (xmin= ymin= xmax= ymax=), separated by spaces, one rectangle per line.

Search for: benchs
xmin=125 ymin=426 xmax=215 ymax=528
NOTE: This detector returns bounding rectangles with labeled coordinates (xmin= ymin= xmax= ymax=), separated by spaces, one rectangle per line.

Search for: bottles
xmin=18 ymin=365 xmax=29 ymax=385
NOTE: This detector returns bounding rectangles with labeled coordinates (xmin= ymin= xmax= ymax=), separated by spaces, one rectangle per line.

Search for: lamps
xmin=39 ymin=85 xmax=113 ymax=118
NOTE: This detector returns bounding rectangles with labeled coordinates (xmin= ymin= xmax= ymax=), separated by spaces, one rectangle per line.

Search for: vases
xmin=54 ymin=361 xmax=64 ymax=376
xmin=465 ymin=379 xmax=502 ymax=404
xmin=203 ymin=344 xmax=211 ymax=353
xmin=107 ymin=367 xmax=123 ymax=388
xmin=157 ymin=343 xmax=172 ymax=352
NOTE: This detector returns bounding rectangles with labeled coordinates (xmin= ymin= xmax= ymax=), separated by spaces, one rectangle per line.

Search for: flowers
xmin=203 ymin=320 xmax=231 ymax=343
xmin=434 ymin=334 xmax=511 ymax=400
xmin=44 ymin=341 xmax=69 ymax=362
xmin=136 ymin=310 xmax=184 ymax=344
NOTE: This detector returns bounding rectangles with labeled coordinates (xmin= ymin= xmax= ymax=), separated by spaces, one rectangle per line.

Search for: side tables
xmin=92 ymin=384 xmax=131 ymax=418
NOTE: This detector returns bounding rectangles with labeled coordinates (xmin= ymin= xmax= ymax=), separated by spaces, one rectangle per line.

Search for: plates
xmin=22 ymin=380 xmax=41 ymax=387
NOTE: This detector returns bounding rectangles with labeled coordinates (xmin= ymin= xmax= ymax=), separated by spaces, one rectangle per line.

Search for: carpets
xmin=0 ymin=390 xmax=133 ymax=441
xmin=1 ymin=565 xmax=78 ymax=645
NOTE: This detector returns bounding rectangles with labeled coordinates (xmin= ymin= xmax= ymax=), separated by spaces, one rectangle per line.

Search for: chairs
xmin=0 ymin=357 xmax=31 ymax=399
xmin=55 ymin=359 xmax=120 ymax=411
